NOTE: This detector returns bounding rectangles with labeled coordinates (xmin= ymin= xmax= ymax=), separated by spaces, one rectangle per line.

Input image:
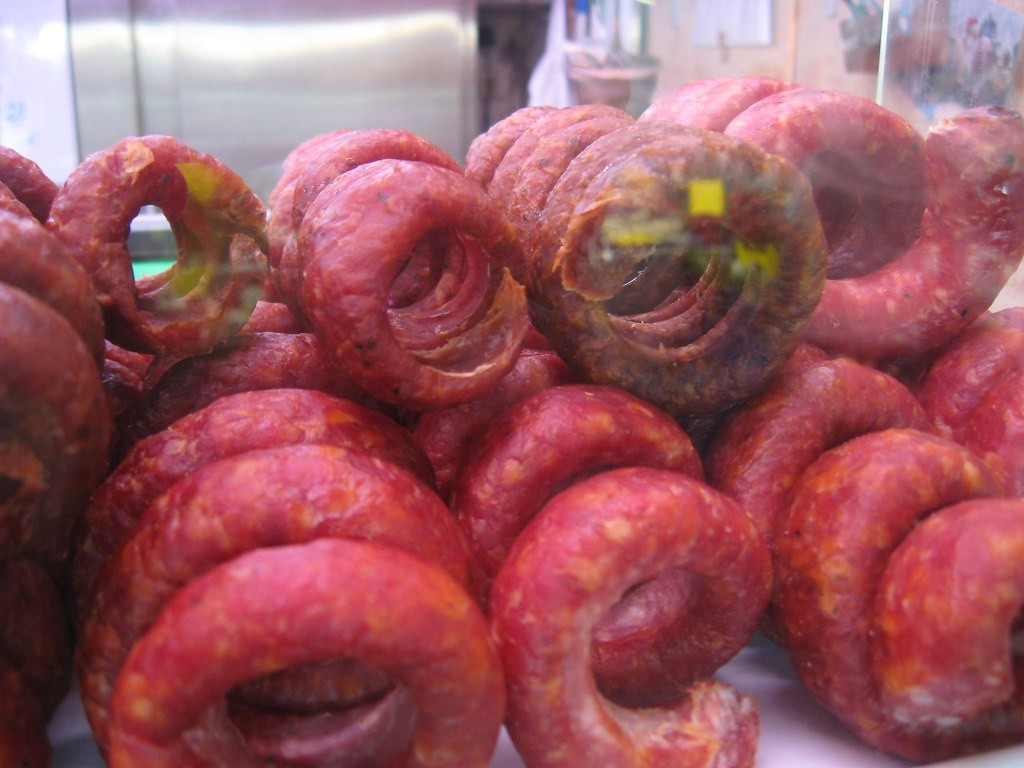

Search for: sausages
xmin=0 ymin=77 xmax=1024 ymax=768
